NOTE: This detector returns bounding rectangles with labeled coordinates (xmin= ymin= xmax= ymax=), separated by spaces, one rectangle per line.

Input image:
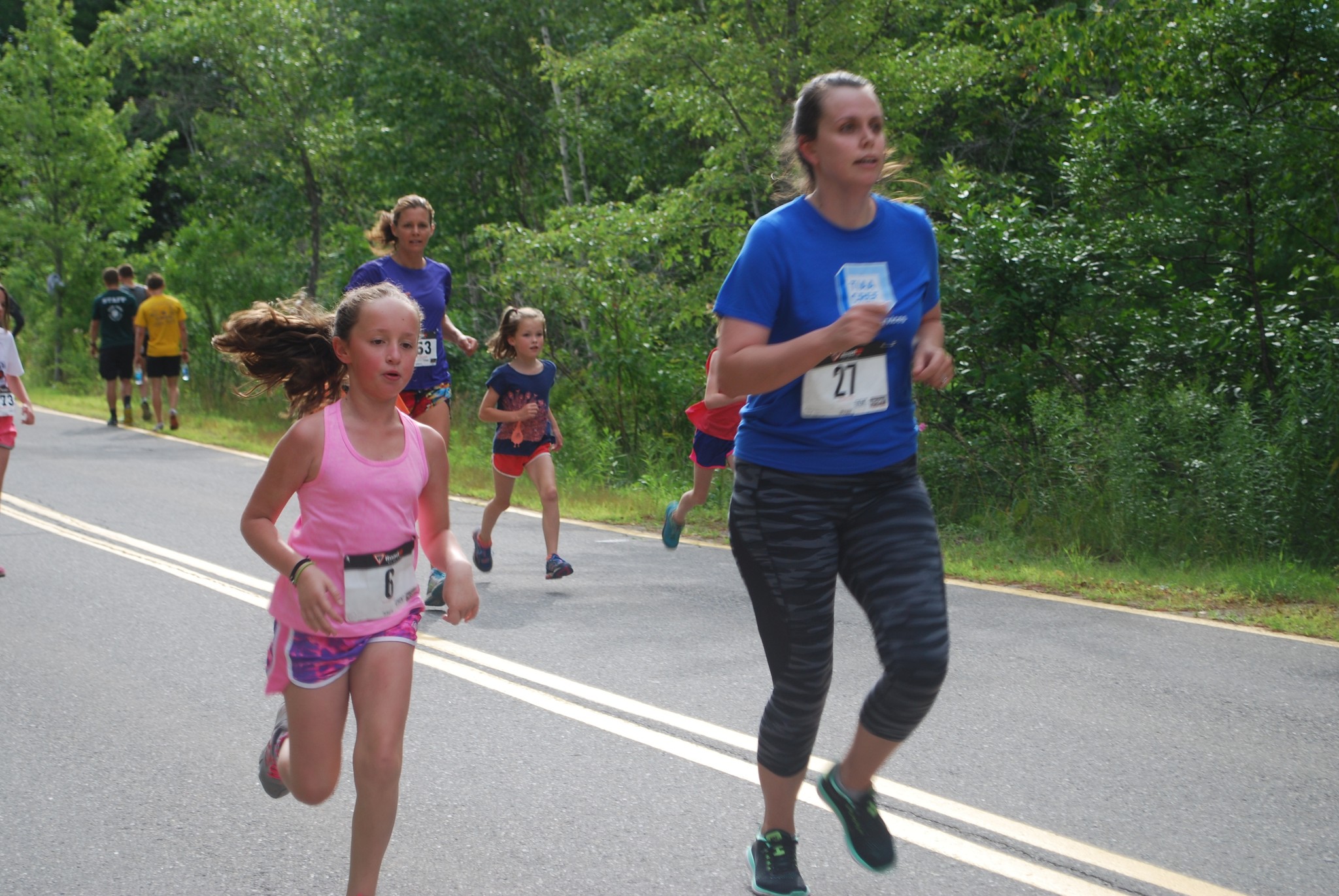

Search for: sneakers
xmin=747 ymin=825 xmax=810 ymax=896
xmin=818 ymin=763 xmax=897 ymax=872
xmin=424 ymin=568 xmax=446 ymax=606
xmin=546 ymin=553 xmax=573 ymax=579
xmin=259 ymin=702 xmax=291 ymax=798
xmin=473 ymin=528 xmax=492 ymax=572
xmin=661 ymin=501 xmax=685 ymax=548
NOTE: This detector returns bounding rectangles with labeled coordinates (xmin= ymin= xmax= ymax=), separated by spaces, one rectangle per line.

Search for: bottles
xmin=135 ymin=367 xmax=143 ymax=385
xmin=182 ymin=362 xmax=190 ymax=381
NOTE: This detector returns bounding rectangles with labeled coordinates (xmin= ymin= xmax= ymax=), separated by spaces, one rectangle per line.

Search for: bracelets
xmin=290 ymin=556 xmax=316 ymax=588
xmin=89 ymin=342 xmax=96 ymax=347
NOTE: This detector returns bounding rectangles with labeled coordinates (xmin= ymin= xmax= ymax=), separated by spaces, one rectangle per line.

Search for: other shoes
xmin=142 ymin=401 xmax=151 ymax=420
xmin=124 ymin=405 xmax=133 ymax=424
xmin=108 ymin=419 xmax=118 ymax=426
xmin=154 ymin=424 xmax=164 ymax=431
xmin=169 ymin=411 xmax=179 ymax=430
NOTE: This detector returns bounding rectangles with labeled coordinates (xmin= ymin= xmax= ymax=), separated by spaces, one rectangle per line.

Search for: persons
xmin=0 ymin=283 xmax=36 ymax=494
xmin=345 ymin=193 xmax=480 ymax=605
xmin=89 ymin=266 xmax=138 ymax=425
xmin=663 ymin=347 xmax=748 ymax=552
xmin=472 ymin=304 xmax=574 ymax=580
xmin=118 ymin=263 xmax=151 ymax=422
xmin=214 ymin=285 xmax=477 ymax=895
xmin=133 ymin=270 xmax=190 ymax=434
xmin=708 ymin=75 xmax=952 ymax=895
